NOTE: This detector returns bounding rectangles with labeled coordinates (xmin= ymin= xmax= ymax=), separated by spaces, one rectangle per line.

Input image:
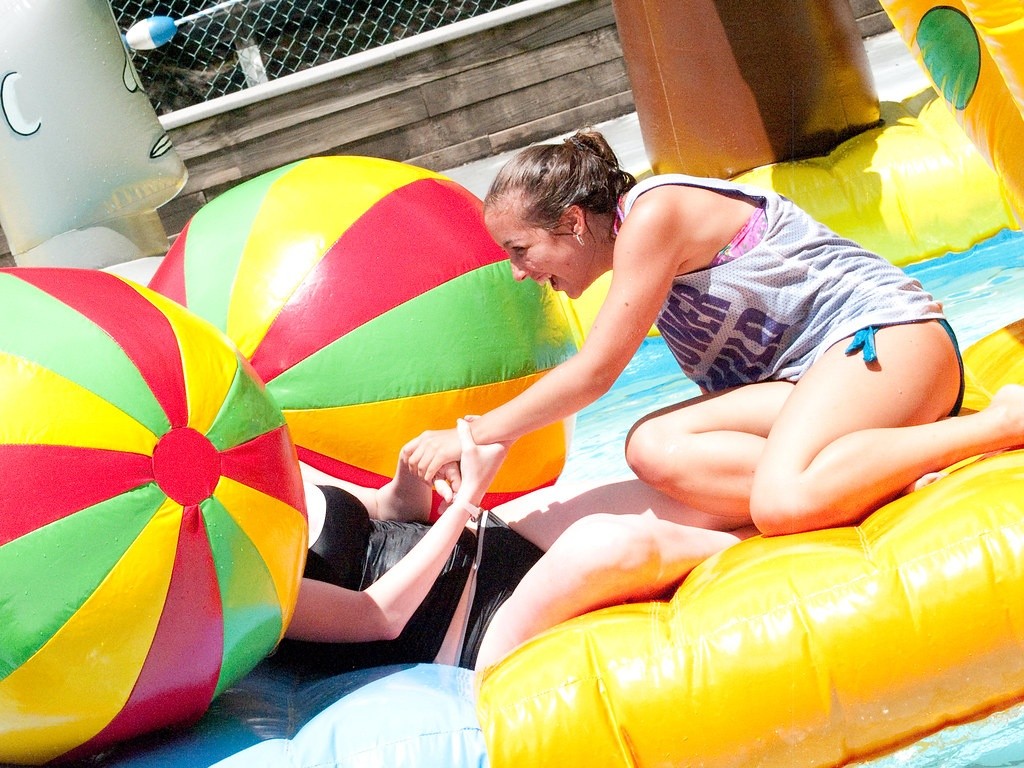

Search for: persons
xmin=267 ymin=412 xmax=764 ymax=671
xmin=401 ymin=130 xmax=1024 ymax=536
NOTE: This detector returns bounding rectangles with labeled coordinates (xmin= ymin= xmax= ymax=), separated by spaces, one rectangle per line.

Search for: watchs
xmin=451 ymin=495 xmax=485 ymax=524
